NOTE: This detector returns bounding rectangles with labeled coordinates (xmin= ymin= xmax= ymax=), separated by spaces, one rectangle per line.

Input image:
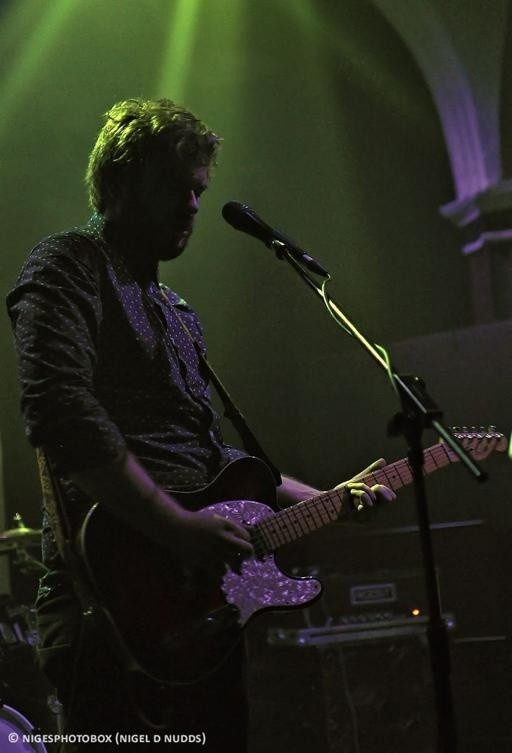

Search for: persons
xmin=4 ymin=99 xmax=398 ymax=752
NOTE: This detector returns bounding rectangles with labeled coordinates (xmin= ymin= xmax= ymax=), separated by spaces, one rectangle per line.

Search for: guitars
xmin=85 ymin=426 xmax=506 ymax=678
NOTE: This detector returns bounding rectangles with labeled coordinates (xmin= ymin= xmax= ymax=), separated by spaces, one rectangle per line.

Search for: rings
xmin=230 ymin=552 xmax=241 ymax=564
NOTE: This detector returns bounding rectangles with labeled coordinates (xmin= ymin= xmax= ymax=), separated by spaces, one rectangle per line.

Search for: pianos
xmin=265 ymin=611 xmax=457 ymax=650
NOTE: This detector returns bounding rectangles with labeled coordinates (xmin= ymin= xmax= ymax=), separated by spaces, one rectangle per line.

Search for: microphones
xmin=221 ymin=200 xmax=331 ymax=280
